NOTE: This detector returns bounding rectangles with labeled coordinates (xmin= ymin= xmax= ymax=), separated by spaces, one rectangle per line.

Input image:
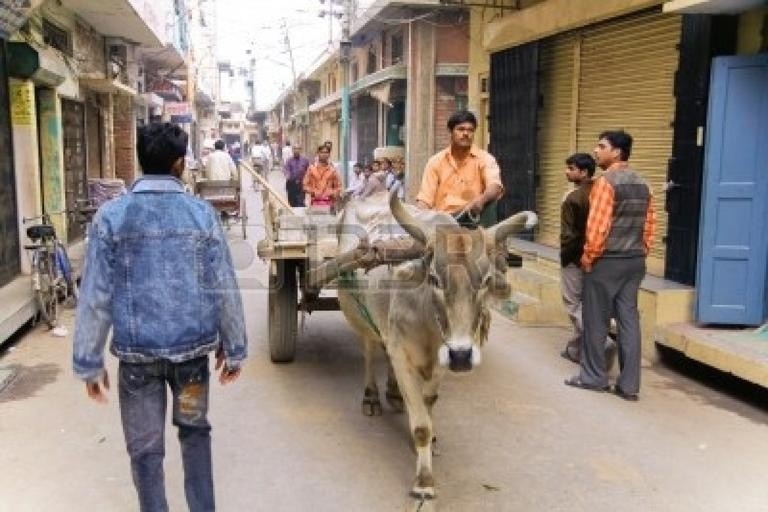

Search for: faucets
xmin=560 ymin=336 xmax=638 ymax=400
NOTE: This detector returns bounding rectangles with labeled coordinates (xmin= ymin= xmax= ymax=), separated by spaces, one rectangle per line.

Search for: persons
xmin=72 ymin=121 xmax=251 ymax=512
xmin=556 ymin=150 xmax=621 ymax=363
xmin=411 ymin=109 xmax=505 ymax=232
xmin=201 ymin=128 xmax=405 ymax=210
xmin=562 ymin=128 xmax=654 ymax=403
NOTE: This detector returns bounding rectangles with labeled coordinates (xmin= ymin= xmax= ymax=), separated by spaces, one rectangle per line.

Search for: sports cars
xmin=193 ymin=164 xmax=250 ymax=243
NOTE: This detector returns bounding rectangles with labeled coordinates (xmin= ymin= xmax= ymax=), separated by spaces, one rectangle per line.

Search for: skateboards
xmin=338 ymin=178 xmax=539 ymax=501
